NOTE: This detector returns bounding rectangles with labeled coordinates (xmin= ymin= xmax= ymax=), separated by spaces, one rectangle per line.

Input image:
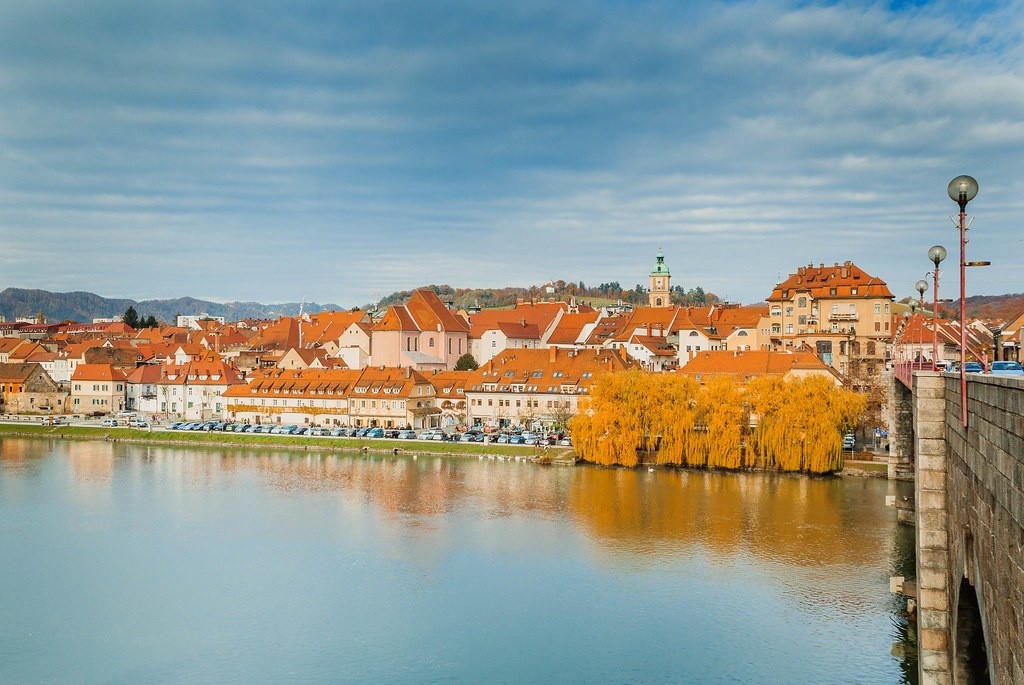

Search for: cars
xmin=935 ymin=361 xmax=1024 ymax=375
xmin=165 ymin=421 xmax=261 ymax=433
xmin=367 ymin=428 xmax=385 ymax=438
xmin=40 ymin=417 xmax=61 ymax=426
xmin=100 ymin=419 xmax=118 ymax=427
xmin=261 ymin=424 xmax=331 ymax=436
xmin=117 ymin=410 xmax=137 ymax=417
xmin=460 ymin=427 xmax=572 ymax=446
xmin=417 ymin=427 xmax=461 ymax=441
xmin=384 ymin=430 xmax=400 ymax=438
xmin=127 ymin=419 xmax=147 ymax=427
xmin=398 ymin=430 xmax=416 ymax=439
xmin=330 ymin=427 xmax=384 ymax=438
xmin=842 ymin=430 xmax=856 ymax=449
xmin=89 ymin=411 xmax=105 ymax=417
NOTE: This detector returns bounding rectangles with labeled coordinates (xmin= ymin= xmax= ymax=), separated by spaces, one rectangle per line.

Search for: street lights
xmin=947 ymin=175 xmax=991 ymax=427
xmin=15 ymin=399 xmax=18 ymax=423
xmin=915 ymin=280 xmax=929 ymax=370
xmin=992 ymin=345 xmax=996 ymax=361
xmin=1012 ymin=338 xmax=1016 ymax=362
xmin=1001 ymin=341 xmax=1004 ymax=361
xmin=463 ymin=395 xmax=468 ymax=432
xmin=893 ymin=299 xmax=918 ymax=389
xmin=928 ymin=245 xmax=947 ymax=371
